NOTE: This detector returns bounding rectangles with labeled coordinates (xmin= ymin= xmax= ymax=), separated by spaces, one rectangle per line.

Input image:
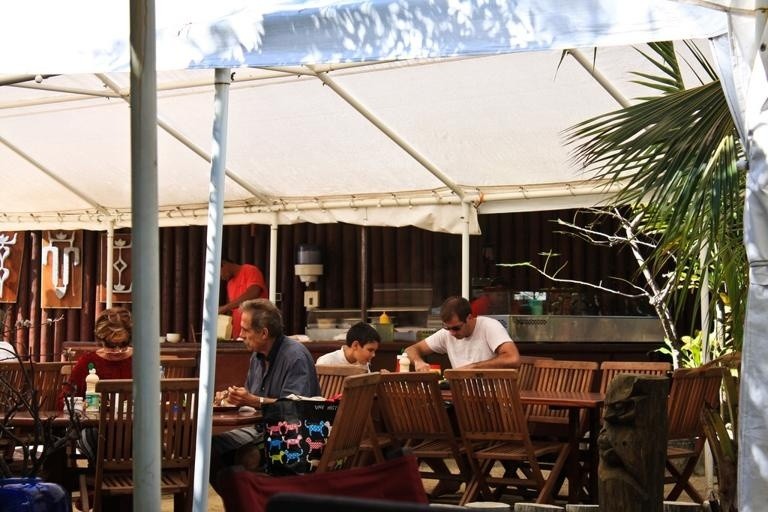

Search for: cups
xmin=529 ymin=299 xmax=544 ymax=315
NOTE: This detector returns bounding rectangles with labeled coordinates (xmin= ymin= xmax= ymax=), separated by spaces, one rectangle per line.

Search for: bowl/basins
xmin=167 ymin=333 xmax=180 ymax=343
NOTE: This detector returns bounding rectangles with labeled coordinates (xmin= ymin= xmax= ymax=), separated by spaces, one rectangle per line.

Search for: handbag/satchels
xmin=257 ymin=397 xmax=341 ymax=477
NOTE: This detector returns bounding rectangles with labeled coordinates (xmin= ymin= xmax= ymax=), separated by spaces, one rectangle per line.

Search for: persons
xmin=468 ymin=276 xmax=526 ymax=314
xmin=632 ymin=236 xmax=680 ymax=362
xmin=207 ymin=298 xmax=323 ymax=512
xmin=316 ymin=320 xmax=381 ymax=374
xmin=217 ymin=255 xmax=269 ymax=339
xmin=0 ymin=341 xmax=16 ymax=360
xmin=401 ymin=295 xmax=521 ymax=392
xmin=530 ymin=271 xmax=605 ymax=361
xmin=51 ymin=307 xmax=133 ymax=410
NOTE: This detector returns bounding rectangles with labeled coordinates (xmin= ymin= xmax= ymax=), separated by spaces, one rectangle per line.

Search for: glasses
xmin=102 ymin=336 xmax=131 ymax=348
xmin=439 ymin=320 xmax=465 ymax=331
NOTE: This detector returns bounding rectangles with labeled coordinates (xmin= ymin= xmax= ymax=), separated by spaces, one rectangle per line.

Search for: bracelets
xmin=258 ymin=396 xmax=266 ymax=408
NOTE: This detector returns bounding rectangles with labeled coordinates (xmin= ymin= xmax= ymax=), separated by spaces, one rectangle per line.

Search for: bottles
xmin=160 ymin=365 xmax=165 ymax=401
xmin=399 ymin=352 xmax=411 ymax=372
xmin=429 ymin=364 xmax=441 ymax=376
xmin=394 ymin=355 xmax=402 ymax=372
xmin=85 ymin=362 xmax=99 ymax=412
xmin=380 ymin=311 xmax=388 ymax=324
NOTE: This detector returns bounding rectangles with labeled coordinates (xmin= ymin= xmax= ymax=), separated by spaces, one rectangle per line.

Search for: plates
xmin=213 ymin=406 xmax=240 ymax=412
